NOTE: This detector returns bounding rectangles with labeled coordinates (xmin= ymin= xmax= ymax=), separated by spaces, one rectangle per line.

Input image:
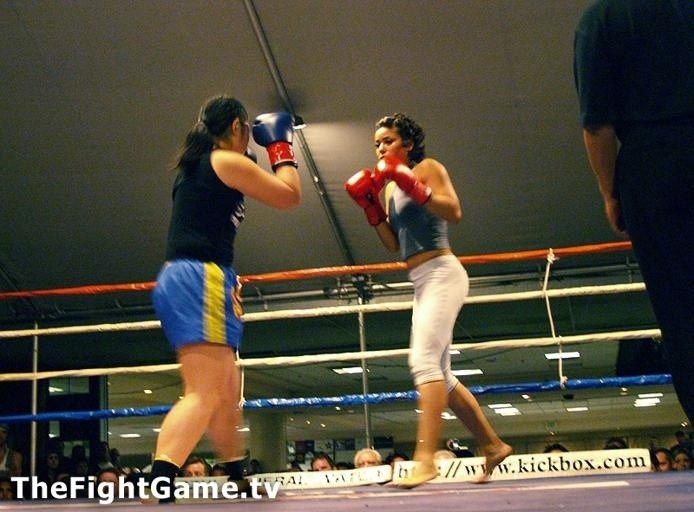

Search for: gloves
xmin=252 ymin=111 xmax=297 ymax=171
xmin=373 ymin=156 xmax=433 ymax=205
xmin=344 ymin=167 xmax=387 ymax=227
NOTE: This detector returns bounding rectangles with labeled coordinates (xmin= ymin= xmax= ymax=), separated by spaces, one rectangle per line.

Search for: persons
xmin=134 ymin=91 xmax=305 ymax=507
xmin=343 ymin=112 xmax=514 ymax=490
xmin=0 ymin=422 xmax=694 ymax=498
xmin=568 ymin=0 xmax=694 ymax=426
